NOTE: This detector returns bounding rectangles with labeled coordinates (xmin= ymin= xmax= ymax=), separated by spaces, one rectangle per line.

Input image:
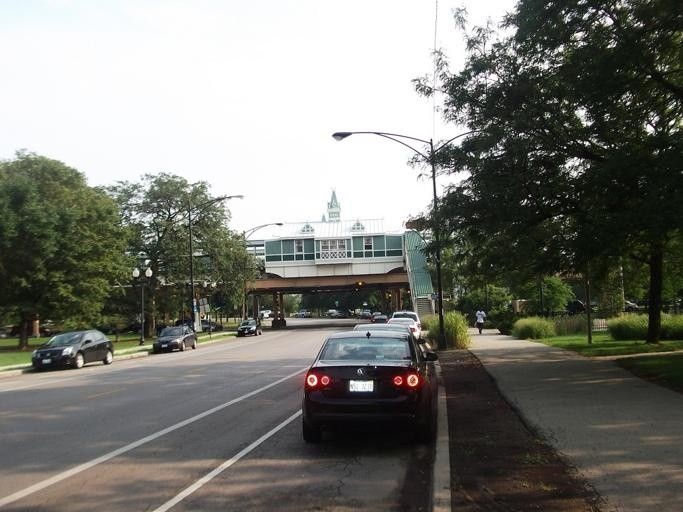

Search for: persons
xmin=473 ymin=307 xmax=486 ymax=334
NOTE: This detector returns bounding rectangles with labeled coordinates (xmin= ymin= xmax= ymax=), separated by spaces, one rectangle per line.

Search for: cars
xmin=151 ymin=325 xmax=197 ymax=354
xmin=236 ymin=319 xmax=262 ymax=337
xmin=300 ymin=308 xmax=440 ymax=446
xmin=567 ymin=300 xmax=585 ymax=314
xmin=177 ymin=320 xmax=219 ymax=332
xmin=260 ymin=309 xmax=312 ymax=319
xmin=625 ymin=300 xmax=638 ymax=312
xmin=30 ymin=328 xmax=112 ymax=372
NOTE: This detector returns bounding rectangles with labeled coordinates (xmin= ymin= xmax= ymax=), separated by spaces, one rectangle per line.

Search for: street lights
xmin=235 ymin=221 xmax=283 ymax=316
xmin=132 ymin=258 xmax=153 ymax=346
xmin=187 ymin=194 xmax=243 ymax=340
xmin=203 ymin=278 xmax=217 ymax=338
xmin=330 ymin=129 xmax=450 ymax=350
